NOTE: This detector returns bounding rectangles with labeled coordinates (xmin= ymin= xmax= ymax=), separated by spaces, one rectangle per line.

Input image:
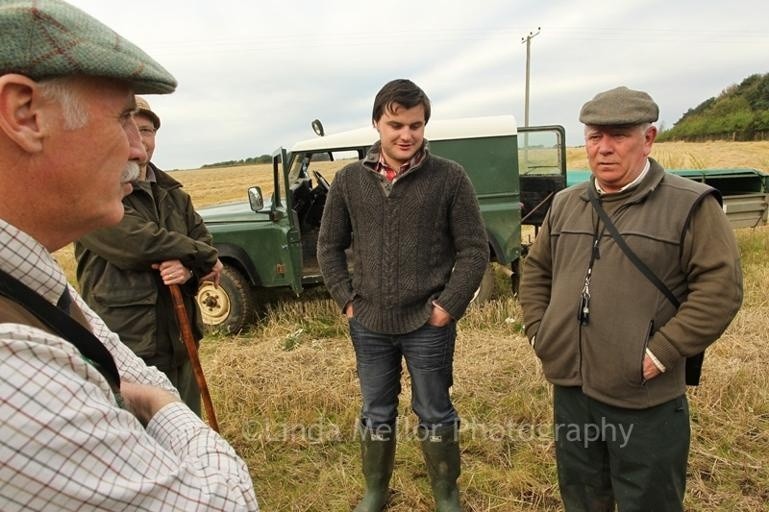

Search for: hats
xmin=132 ymin=96 xmax=160 ymax=131
xmin=579 ymin=87 xmax=661 ymax=127
xmin=0 ymin=0 xmax=177 ymax=94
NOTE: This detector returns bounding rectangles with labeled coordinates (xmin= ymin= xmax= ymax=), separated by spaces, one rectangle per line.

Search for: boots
xmin=352 ymin=419 xmax=395 ymax=512
xmin=416 ymin=423 xmax=460 ymax=512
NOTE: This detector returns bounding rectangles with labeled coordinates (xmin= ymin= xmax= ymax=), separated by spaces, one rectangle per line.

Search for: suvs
xmin=192 ymin=114 xmax=568 ymax=338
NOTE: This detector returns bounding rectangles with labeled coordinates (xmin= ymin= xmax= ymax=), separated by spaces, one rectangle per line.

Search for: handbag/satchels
xmin=683 ymin=350 xmax=704 ymax=386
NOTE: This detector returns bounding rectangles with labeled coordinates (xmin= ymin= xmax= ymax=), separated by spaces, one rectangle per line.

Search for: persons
xmin=72 ymin=94 xmax=225 ymax=428
xmin=317 ymin=81 xmax=490 ymax=512
xmin=520 ymin=86 xmax=744 ymax=512
xmin=1 ymin=1 xmax=260 ymax=511
